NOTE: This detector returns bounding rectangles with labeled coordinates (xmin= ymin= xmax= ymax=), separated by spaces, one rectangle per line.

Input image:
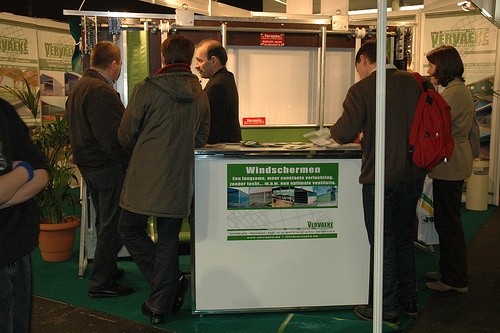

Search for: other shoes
xmin=426 ymin=271 xmax=442 ymax=279
xmin=426 ymin=280 xmax=468 ymax=293
xmin=354 ymin=306 xmax=400 ymax=325
xmin=398 ymin=296 xmax=418 ymax=319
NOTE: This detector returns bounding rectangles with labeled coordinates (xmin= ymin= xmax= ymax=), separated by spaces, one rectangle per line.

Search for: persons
xmin=65 ymin=41 xmax=242 ymax=325
xmin=330 ymin=42 xmax=475 ymax=324
xmin=0 ymin=97 xmax=51 ymax=332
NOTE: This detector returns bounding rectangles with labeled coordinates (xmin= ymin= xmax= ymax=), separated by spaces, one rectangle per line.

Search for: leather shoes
xmin=88 ymin=279 xmax=134 ymax=297
xmin=172 ymin=272 xmax=191 ymax=313
xmin=105 ymin=268 xmax=124 ymax=277
xmin=141 ymin=302 xmax=163 ymax=324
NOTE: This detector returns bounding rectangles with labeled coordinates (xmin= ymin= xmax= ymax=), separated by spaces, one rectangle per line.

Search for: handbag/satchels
xmin=468 ymin=109 xmax=480 ymax=158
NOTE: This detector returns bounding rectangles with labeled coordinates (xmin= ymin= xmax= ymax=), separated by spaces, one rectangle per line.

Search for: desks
xmin=191 ymin=141 xmax=370 ymax=316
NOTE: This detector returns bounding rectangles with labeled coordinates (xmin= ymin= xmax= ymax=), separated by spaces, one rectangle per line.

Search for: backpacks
xmin=408 ymin=72 xmax=454 ymax=169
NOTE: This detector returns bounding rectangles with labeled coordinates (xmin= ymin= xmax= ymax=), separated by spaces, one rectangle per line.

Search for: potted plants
xmin=0 ymin=71 xmax=82 ymax=261
